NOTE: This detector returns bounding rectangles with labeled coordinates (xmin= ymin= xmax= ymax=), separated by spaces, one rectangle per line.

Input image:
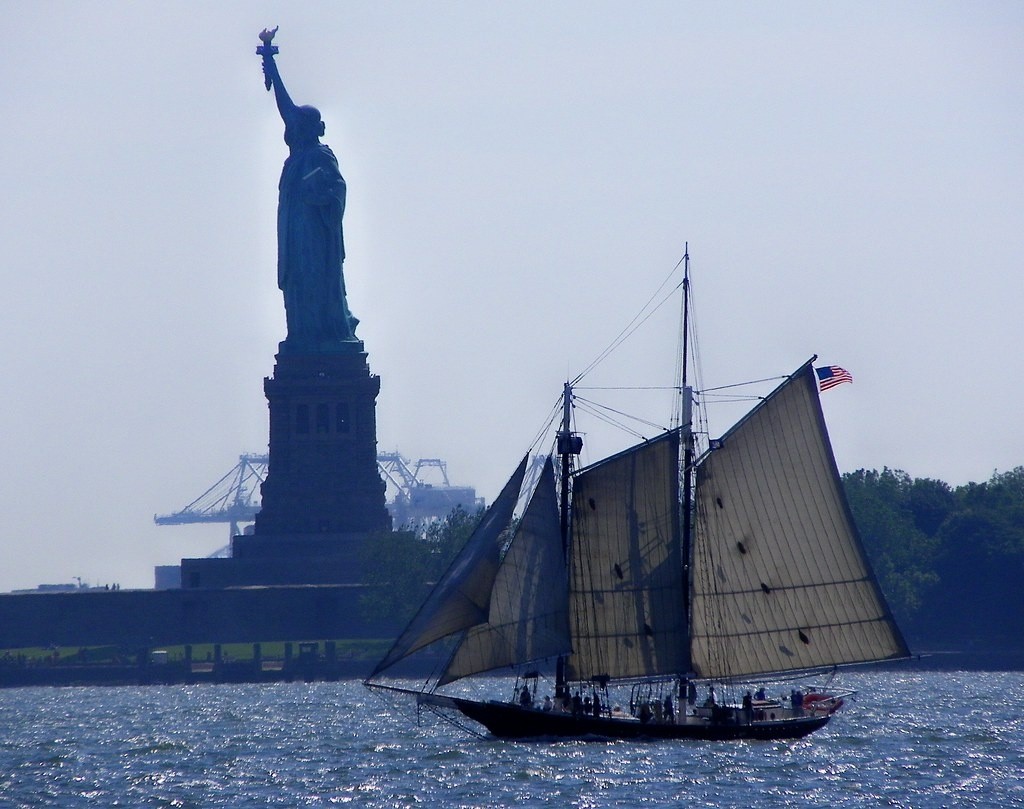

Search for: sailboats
xmin=361 ymin=239 xmax=932 ymax=743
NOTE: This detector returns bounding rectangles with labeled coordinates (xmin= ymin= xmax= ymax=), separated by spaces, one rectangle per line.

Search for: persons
xmin=742 ymin=690 xmax=754 ymax=726
xmin=543 ymin=695 xmax=552 ymax=711
xmin=704 ymin=687 xmax=717 ymax=707
xmin=520 ymin=686 xmax=531 ymax=707
xmin=262 ymin=59 xmax=359 ymax=342
xmin=791 ymin=689 xmax=804 ymax=706
xmin=754 ymin=688 xmax=766 ymax=701
xmin=562 ymin=686 xmax=601 ymax=715
xmin=662 ymin=695 xmax=674 ymax=720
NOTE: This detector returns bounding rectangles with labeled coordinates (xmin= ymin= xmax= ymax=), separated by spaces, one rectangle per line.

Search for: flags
xmin=815 ymin=365 xmax=853 ymax=392
